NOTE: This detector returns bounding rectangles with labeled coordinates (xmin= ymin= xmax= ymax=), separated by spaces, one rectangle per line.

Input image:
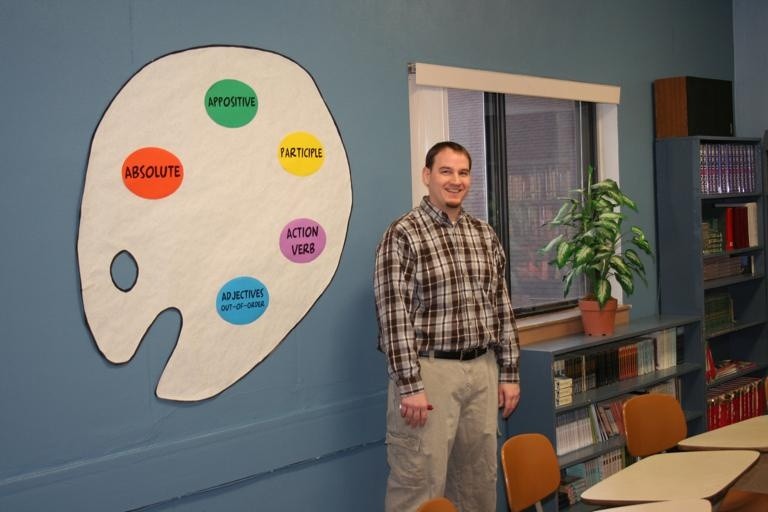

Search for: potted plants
xmin=536 ymin=162 xmax=656 ymax=339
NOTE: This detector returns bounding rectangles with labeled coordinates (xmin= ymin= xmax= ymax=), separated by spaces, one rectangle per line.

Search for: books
xmin=508 ymin=161 xmax=576 ymax=281
xmin=701 ymin=143 xmax=758 ymax=282
xmin=555 ymin=323 xmax=687 ymax=506
xmin=707 ymin=377 xmax=768 ymax=431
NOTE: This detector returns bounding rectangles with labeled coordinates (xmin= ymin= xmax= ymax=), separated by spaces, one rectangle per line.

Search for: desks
xmin=593 ymin=500 xmax=712 ymax=512
xmin=678 ymin=415 xmax=768 ymax=454
xmin=581 ymin=450 xmax=760 ymax=506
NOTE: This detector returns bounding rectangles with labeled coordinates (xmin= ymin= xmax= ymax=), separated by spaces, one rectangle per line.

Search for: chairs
xmin=621 ymin=393 xmax=768 ymax=512
xmin=502 ymin=433 xmax=561 ymax=511
xmin=416 ymin=498 xmax=457 ymax=511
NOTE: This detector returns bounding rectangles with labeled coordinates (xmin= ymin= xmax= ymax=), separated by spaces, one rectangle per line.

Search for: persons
xmin=374 ymin=142 xmax=521 ymax=512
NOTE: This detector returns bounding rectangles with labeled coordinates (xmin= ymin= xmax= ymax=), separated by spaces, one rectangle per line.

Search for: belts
xmin=418 ymin=346 xmax=487 ymax=360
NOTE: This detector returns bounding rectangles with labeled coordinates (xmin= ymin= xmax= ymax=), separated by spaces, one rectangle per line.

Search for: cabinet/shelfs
xmin=506 ymin=317 xmax=706 ymax=511
xmin=654 ymin=135 xmax=768 ymax=433
xmin=655 ymin=76 xmax=734 ymax=137
